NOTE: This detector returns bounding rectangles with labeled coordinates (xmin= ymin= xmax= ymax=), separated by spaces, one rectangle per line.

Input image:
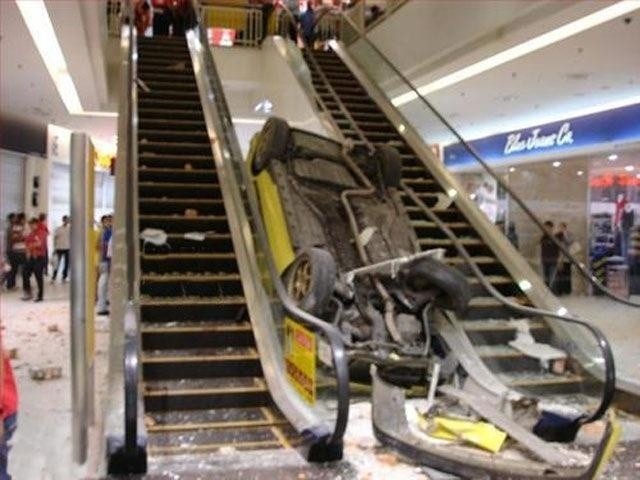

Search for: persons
xmin=1 ymin=322 xmax=19 ymax=480
xmin=300 ymin=0 xmax=317 ymax=49
xmin=539 ymin=221 xmax=557 ymax=294
xmin=554 ymin=221 xmax=575 ymax=294
xmin=0 ymin=213 xmax=113 ymax=316
xmin=609 ymin=190 xmax=633 ymax=262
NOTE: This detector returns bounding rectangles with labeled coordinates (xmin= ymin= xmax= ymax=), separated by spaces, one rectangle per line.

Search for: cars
xmin=243 ymin=117 xmax=473 ymax=397
